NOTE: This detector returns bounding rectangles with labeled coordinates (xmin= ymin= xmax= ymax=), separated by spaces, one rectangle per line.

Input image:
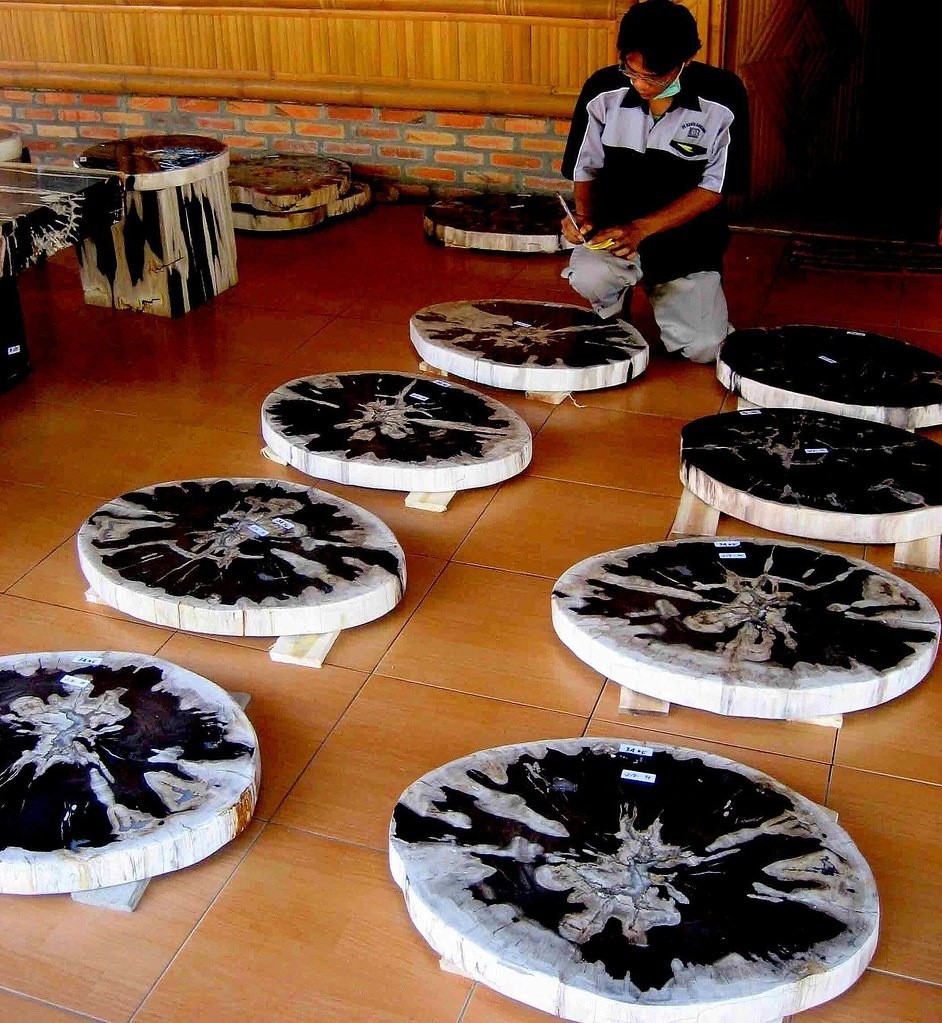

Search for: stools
xmin=73 ymin=132 xmax=238 ymax=319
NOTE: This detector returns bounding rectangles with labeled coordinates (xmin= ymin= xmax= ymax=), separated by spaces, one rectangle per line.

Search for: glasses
xmin=618 ymin=52 xmax=685 ymax=87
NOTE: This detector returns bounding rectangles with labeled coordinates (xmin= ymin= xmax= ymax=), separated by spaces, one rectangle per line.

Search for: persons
xmin=561 ymin=1 xmax=751 ymax=365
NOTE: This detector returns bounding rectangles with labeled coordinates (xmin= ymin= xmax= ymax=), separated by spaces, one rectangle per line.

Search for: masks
xmin=630 ymin=61 xmax=687 ymax=100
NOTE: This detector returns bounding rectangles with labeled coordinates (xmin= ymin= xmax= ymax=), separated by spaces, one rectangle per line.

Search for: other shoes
xmin=614 ymin=286 xmax=634 ymax=325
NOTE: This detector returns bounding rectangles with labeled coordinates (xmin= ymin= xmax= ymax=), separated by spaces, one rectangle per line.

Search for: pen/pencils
xmin=554 ymin=190 xmax=588 ymax=244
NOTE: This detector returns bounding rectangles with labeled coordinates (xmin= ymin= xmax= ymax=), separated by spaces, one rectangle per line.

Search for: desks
xmin=0 ymin=161 xmax=123 ymax=395
